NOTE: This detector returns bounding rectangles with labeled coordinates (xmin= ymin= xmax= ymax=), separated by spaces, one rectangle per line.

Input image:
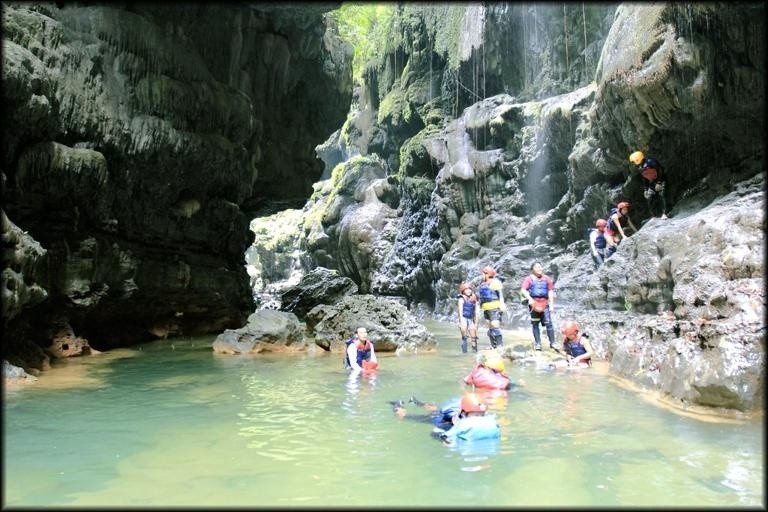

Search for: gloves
xmin=655 ymin=184 xmax=662 ymax=191
xmin=644 ymin=191 xmax=652 ymax=199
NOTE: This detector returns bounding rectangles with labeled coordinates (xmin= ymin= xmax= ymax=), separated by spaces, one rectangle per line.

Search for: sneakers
xmin=661 ymin=214 xmax=668 ymax=220
xmin=535 ymin=343 xmax=542 ymax=350
xmin=550 ymin=341 xmax=560 ymax=350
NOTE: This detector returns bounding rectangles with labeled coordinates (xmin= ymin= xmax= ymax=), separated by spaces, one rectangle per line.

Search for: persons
xmin=561 ymin=320 xmax=594 ymax=368
xmin=458 ymin=282 xmax=480 ymax=353
xmin=345 ymin=328 xmax=377 ymax=379
xmin=630 ymin=151 xmax=668 ymax=220
xmin=479 ymin=266 xmax=505 ymax=349
xmin=589 ymin=219 xmax=608 ymax=268
xmin=521 ymin=261 xmax=562 ymax=353
xmin=390 ymin=395 xmax=466 ymax=431
xmin=435 ymin=393 xmax=502 ymax=453
xmin=603 ymin=201 xmax=638 ymax=249
xmin=465 ymin=353 xmax=512 ymax=434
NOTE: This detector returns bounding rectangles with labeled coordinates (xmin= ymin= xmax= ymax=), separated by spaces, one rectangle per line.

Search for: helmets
xmin=561 ymin=321 xmax=579 ymax=335
xmin=460 ymin=283 xmax=473 ymax=293
xmin=533 ymin=298 xmax=546 ymax=312
xmin=363 ymin=359 xmax=378 ymax=370
xmin=630 ymin=151 xmax=644 ymax=165
xmin=483 ymin=267 xmax=496 ymax=276
xmin=460 ymin=394 xmax=487 ymax=412
xmin=596 ymin=218 xmax=607 ymax=227
xmin=618 ymin=202 xmax=631 ymax=211
